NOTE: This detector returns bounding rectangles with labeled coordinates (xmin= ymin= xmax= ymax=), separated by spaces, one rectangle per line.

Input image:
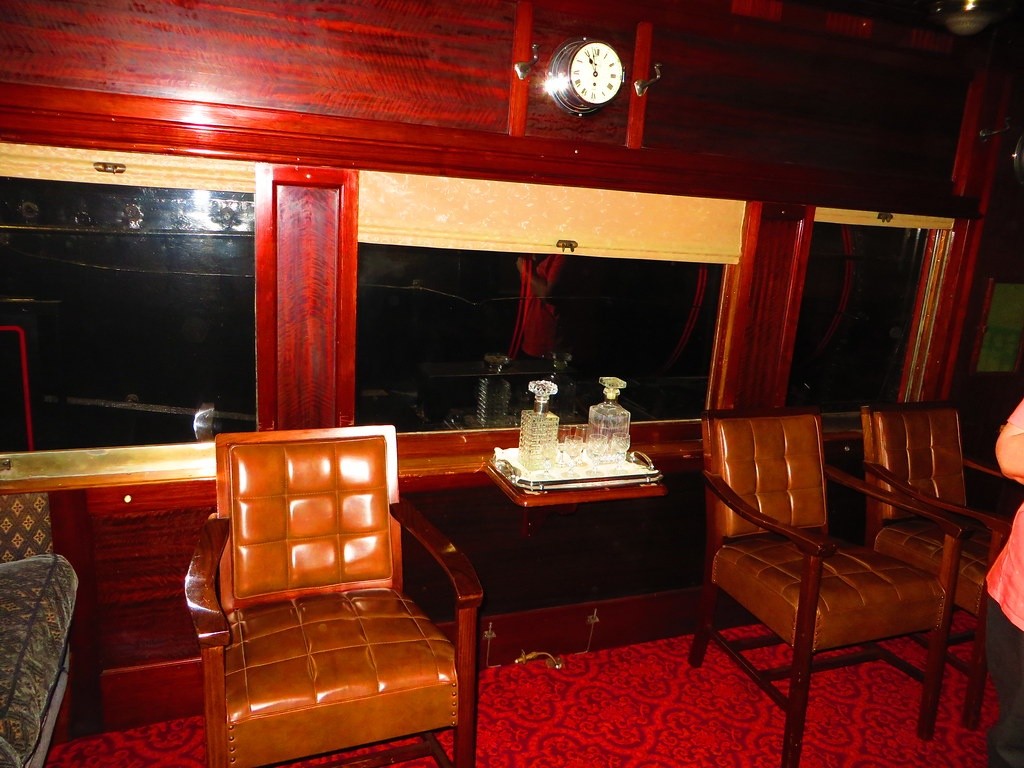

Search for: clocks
xmin=544 ymin=37 xmax=627 ymax=116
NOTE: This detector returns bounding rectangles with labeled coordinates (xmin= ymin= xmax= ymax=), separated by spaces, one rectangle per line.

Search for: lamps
xmin=930 ymin=0 xmax=1004 ymax=35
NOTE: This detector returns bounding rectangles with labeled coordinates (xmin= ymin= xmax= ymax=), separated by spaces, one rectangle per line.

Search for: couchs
xmin=0 ymin=489 xmax=104 ymax=768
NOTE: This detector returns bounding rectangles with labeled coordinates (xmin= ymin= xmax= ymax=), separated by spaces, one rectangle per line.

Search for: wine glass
xmin=536 ymin=432 xmax=630 ymax=480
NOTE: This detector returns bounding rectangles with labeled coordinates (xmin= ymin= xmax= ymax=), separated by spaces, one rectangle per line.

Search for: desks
xmin=483 ymin=458 xmax=667 ymax=537
xmin=414 ymin=361 xmax=583 ymax=424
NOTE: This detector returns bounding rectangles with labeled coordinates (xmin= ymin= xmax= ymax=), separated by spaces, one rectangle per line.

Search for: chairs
xmin=184 ymin=424 xmax=485 ymax=768
xmin=689 ymin=407 xmax=976 ymax=768
xmin=859 ymin=403 xmax=1018 ymax=729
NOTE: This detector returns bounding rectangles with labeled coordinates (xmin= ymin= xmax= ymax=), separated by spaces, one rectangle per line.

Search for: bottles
xmin=548 ymin=353 xmax=578 ymax=422
xmin=519 ymin=380 xmax=560 ymax=470
xmin=587 ymin=376 xmax=631 ymax=465
xmin=476 ymin=353 xmax=512 ymax=428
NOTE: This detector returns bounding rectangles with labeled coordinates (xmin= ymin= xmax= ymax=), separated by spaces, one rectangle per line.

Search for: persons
xmin=507 ymin=253 xmax=571 ymax=402
xmin=985 ymin=397 xmax=1024 ymax=768
